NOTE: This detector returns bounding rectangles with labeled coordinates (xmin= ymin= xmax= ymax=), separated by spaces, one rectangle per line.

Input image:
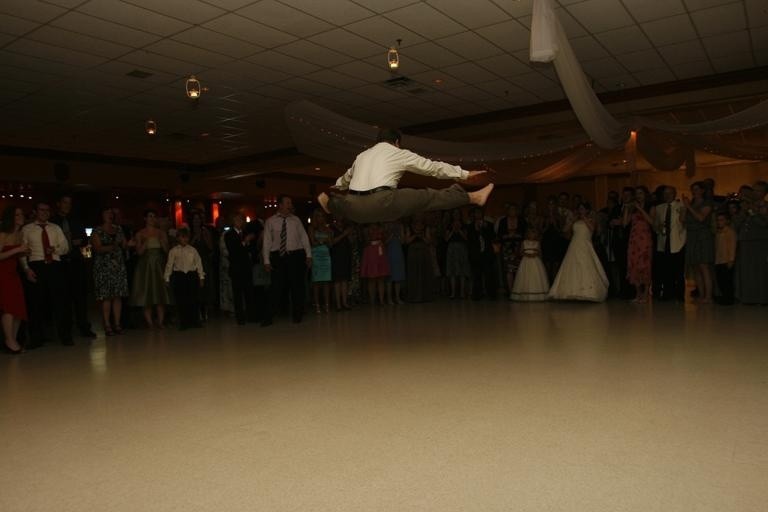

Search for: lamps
xmin=186 ymin=74 xmax=201 ymax=98
xmin=387 ymin=46 xmax=399 ymax=68
xmin=145 ymin=119 xmax=156 ymax=136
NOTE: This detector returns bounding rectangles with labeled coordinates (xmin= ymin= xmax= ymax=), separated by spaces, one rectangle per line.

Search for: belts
xmin=348 ymin=186 xmax=391 ymax=195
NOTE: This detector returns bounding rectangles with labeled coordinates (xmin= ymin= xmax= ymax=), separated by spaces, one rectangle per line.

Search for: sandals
xmin=313 ymin=304 xmax=321 ymax=315
xmin=104 ymin=326 xmax=115 ymax=336
xmin=323 ymin=304 xmax=329 ymax=314
xmin=112 ymin=324 xmax=126 ymax=335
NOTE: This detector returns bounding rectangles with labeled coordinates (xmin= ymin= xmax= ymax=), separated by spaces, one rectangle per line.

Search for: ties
xmin=38 ymin=223 xmax=52 ymax=261
xmin=279 ymin=216 xmax=286 ymax=257
xmin=664 ymin=204 xmax=671 ymax=227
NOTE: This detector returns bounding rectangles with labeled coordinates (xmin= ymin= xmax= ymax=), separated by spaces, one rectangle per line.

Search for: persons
xmin=0 ymin=179 xmax=768 ymax=355
xmin=314 ymin=127 xmax=495 ymax=225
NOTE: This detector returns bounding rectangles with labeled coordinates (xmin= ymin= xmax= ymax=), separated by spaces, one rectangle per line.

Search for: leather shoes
xmin=342 ymin=304 xmax=351 ymax=310
xmin=3 ymin=338 xmax=26 ymax=353
xmin=24 ymin=343 xmax=41 ymax=349
xmin=76 ymin=320 xmax=90 ymax=330
xmin=63 ymin=337 xmax=74 ymax=346
xmin=335 ymin=304 xmax=342 ymax=312
